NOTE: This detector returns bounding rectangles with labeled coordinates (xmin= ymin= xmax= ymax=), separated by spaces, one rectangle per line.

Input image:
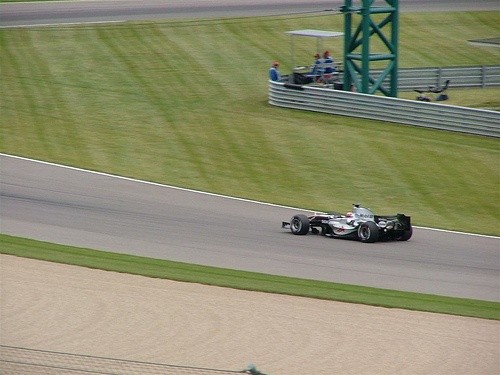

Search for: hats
xmin=314 ymin=54 xmax=320 ymax=57
xmin=273 ymin=62 xmax=279 ymax=66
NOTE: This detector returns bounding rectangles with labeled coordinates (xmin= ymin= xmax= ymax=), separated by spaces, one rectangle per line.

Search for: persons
xmin=268 ymin=61 xmax=281 ymax=83
xmin=347 ymin=204 xmax=361 ymax=218
xmin=322 ymin=51 xmax=336 ymax=82
xmin=312 ymin=52 xmax=323 ymax=84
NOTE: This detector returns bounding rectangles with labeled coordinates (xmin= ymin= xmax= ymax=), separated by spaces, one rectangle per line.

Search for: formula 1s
xmin=282 ymin=203 xmax=413 ymax=244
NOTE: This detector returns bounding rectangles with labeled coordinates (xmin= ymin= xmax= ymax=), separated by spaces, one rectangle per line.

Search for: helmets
xmin=346 ymin=212 xmax=353 ymax=218
